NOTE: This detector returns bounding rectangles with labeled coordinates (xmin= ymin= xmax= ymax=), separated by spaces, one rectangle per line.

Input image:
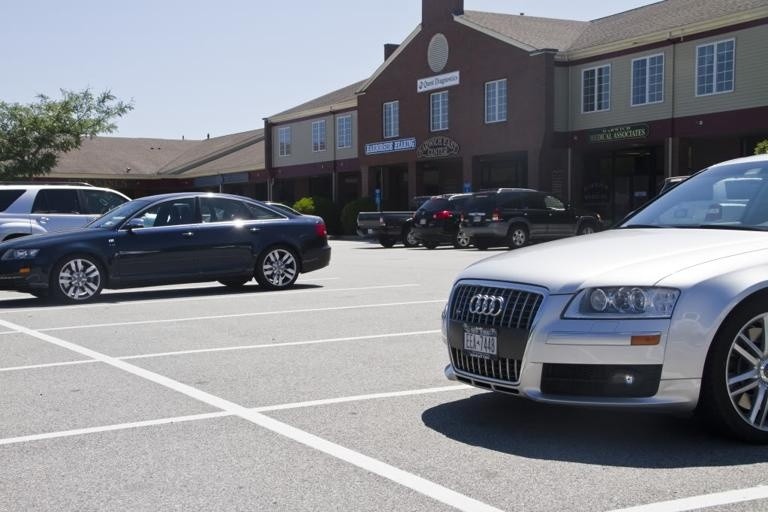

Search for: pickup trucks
xmin=354 ymin=195 xmax=432 ymax=247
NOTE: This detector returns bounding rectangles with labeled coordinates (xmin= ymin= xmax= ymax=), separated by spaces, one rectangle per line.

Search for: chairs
xmin=166 ymin=206 xmax=256 ymax=224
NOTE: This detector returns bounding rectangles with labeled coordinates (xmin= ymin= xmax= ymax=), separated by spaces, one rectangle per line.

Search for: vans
xmin=658 ymin=176 xmax=764 ymax=228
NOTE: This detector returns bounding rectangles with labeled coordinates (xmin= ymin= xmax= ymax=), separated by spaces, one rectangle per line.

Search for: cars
xmin=438 ymin=153 xmax=768 ymax=447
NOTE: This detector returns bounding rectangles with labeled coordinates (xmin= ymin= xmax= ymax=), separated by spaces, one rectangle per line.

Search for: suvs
xmin=415 ymin=192 xmax=472 ymax=248
xmin=456 ymin=186 xmax=602 ymax=248
